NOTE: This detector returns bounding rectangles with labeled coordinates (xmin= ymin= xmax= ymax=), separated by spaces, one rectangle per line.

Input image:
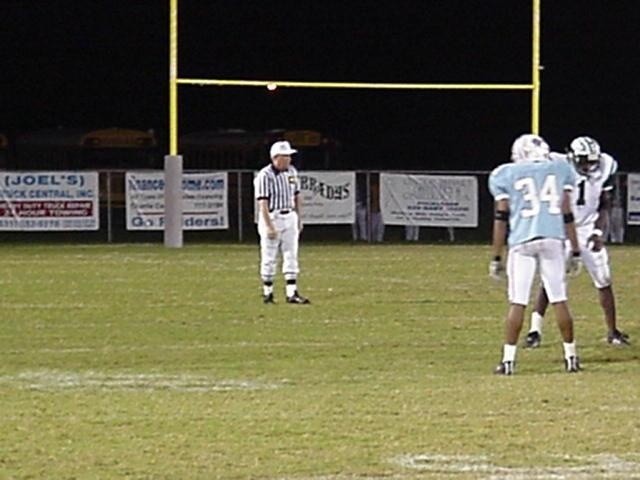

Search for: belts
xmin=280 ymin=210 xmax=290 ymax=214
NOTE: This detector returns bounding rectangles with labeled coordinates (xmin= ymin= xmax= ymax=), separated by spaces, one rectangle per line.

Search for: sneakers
xmin=607 ymin=329 xmax=629 ymax=347
xmin=523 ymin=331 xmax=541 ymax=350
xmin=564 ymin=356 xmax=582 ymax=373
xmin=263 ymin=293 xmax=275 ymax=305
xmin=285 ymin=291 xmax=309 ymax=304
xmin=494 ymin=361 xmax=515 ymax=375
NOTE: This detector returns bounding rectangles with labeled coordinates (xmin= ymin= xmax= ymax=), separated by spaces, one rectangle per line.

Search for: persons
xmin=487 ymin=133 xmax=583 ymax=374
xmin=252 ymin=140 xmax=311 ymax=304
xmin=521 ymin=135 xmax=632 ymax=349
xmin=350 ymin=174 xmax=456 ymax=242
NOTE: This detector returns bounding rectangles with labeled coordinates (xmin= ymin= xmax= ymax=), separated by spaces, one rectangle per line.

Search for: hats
xmin=270 ymin=141 xmax=297 ymax=159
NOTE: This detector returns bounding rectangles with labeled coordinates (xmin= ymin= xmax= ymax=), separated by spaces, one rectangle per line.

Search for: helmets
xmin=510 ymin=133 xmax=550 ymax=164
xmin=570 ymin=135 xmax=602 ymax=177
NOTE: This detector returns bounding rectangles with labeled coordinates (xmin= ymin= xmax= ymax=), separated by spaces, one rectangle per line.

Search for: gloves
xmin=488 ymin=261 xmax=506 ymax=281
xmin=568 ymin=257 xmax=583 ymax=279
